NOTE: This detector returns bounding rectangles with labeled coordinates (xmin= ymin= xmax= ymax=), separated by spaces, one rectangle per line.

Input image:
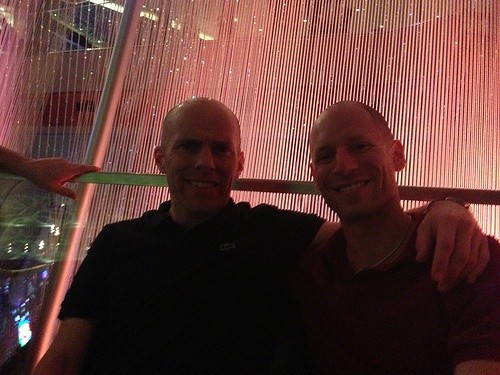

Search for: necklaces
xmin=347 ymin=215 xmax=411 ymax=272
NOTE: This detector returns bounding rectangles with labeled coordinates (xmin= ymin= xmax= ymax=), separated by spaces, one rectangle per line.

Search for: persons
xmin=256 ymin=101 xmax=500 ymax=375
xmin=32 ymin=97 xmax=488 ymax=375
xmin=0 ymin=146 xmax=101 ymax=199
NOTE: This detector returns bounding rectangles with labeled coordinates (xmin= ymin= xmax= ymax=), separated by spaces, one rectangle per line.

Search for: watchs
xmin=426 ymin=197 xmax=471 ymax=212
